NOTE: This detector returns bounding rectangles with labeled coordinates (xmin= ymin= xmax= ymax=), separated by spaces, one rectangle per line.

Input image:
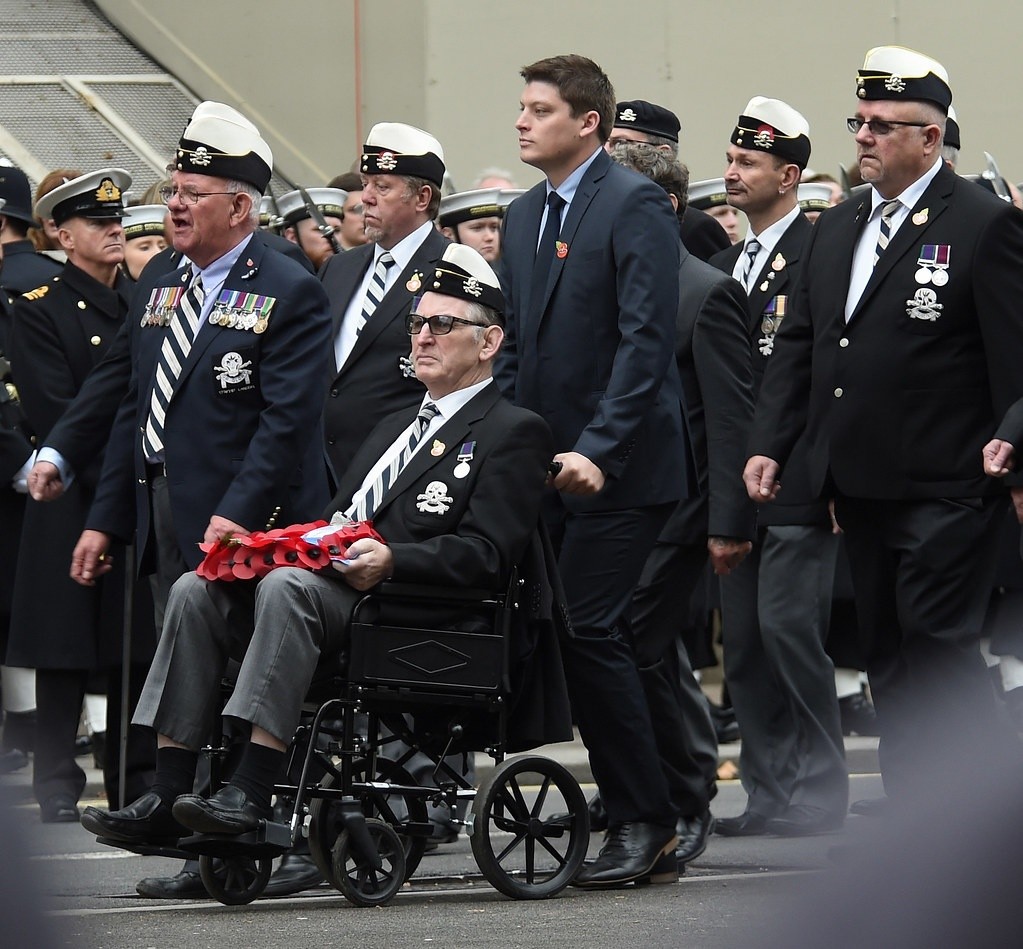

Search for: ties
xmin=522 ymin=190 xmax=568 ymax=360
xmin=139 ymin=269 xmax=206 ymax=463
xmin=873 ymin=199 xmax=903 ymax=270
xmin=355 ymin=252 xmax=395 ymax=340
xmin=738 ymin=239 xmax=762 ymax=293
xmin=349 ymin=402 xmax=440 ymax=524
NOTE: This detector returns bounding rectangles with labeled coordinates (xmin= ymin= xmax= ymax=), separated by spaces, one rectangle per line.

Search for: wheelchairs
xmin=94 ymin=462 xmax=592 ymax=910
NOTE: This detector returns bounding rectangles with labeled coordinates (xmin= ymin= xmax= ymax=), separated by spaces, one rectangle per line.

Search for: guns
xmin=982 ymin=149 xmax=1013 ymax=205
xmin=295 ymin=183 xmax=347 ymax=256
xmin=837 ymin=159 xmax=855 ymax=204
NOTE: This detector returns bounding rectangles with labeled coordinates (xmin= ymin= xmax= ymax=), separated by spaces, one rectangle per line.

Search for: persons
xmin=83 ymin=243 xmax=550 ymax=844
xmin=0 ymin=45 xmax=1023 ymax=898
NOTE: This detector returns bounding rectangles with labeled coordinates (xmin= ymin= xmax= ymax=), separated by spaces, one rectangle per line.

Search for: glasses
xmin=846 ymin=116 xmax=929 ymax=136
xmin=159 ymin=185 xmax=236 ymax=205
xmin=404 ymin=313 xmax=489 ymax=336
xmin=604 ymin=139 xmax=663 ymax=154
xmin=348 ymin=203 xmax=364 ymax=216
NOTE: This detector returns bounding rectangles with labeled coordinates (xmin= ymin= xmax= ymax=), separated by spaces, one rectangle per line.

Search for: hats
xmin=855 ymin=45 xmax=963 ymax=150
xmin=685 ymin=95 xmax=833 ymax=212
xmin=613 ymin=99 xmax=681 ymax=143
xmin=360 ymin=121 xmax=446 ymax=190
xmin=425 ymin=241 xmax=506 ymax=315
xmin=437 ymin=188 xmax=536 ymax=230
xmin=0 ymin=99 xmax=347 ymax=239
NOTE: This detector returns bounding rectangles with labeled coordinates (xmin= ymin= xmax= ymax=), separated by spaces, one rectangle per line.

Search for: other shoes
xmin=705 ymin=661 xmax=1022 ymax=817
xmin=399 ymin=816 xmax=459 ymax=851
xmin=0 ymin=733 xmax=105 ymax=822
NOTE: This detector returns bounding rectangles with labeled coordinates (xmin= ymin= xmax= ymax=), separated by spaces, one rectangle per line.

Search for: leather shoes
xmin=546 ymin=790 xmax=611 ymax=833
xmin=764 ymin=804 xmax=844 ymax=834
xmin=135 ymin=871 xmax=214 ymax=900
xmin=712 ymin=810 xmax=768 ymax=837
xmin=171 ymin=783 xmax=276 ymax=836
xmin=576 ymin=816 xmax=680 ymax=887
xmin=258 ymin=852 xmax=322 ymax=897
xmin=82 ymin=792 xmax=195 ymax=844
xmin=674 ymin=807 xmax=717 ymax=863
